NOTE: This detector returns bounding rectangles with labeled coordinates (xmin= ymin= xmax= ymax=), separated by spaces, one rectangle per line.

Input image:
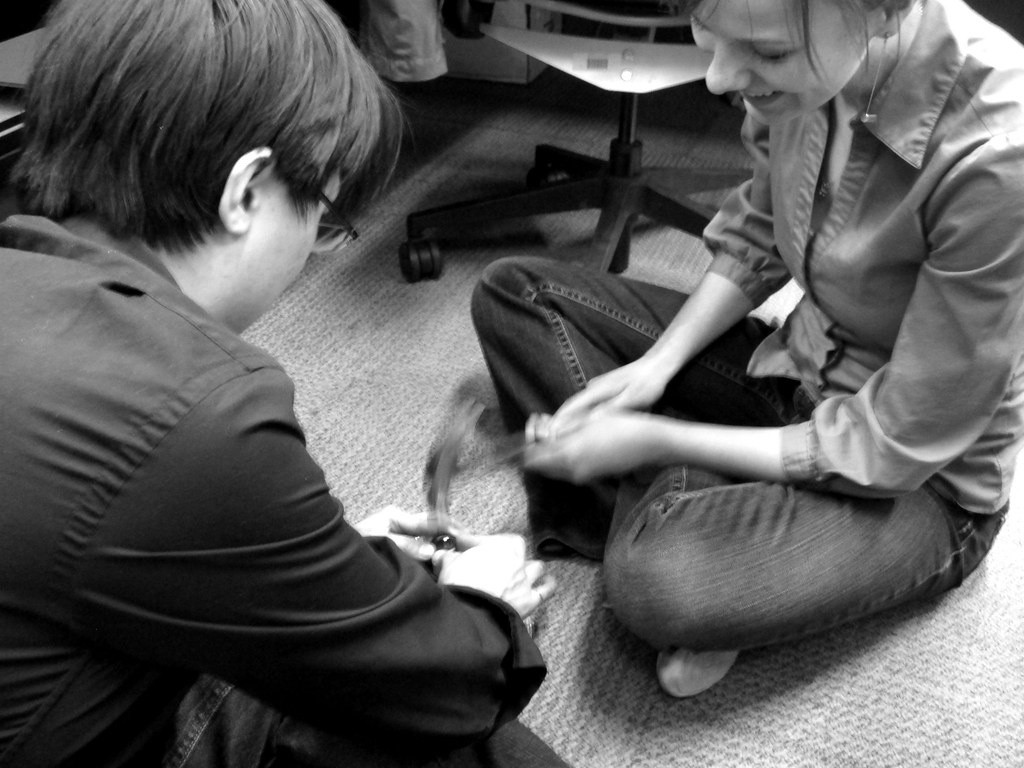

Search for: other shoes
xmin=656 ymin=644 xmax=740 ymax=698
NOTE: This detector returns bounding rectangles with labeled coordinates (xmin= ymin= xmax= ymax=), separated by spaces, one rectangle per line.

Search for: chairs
xmin=398 ymin=0 xmax=718 ymax=283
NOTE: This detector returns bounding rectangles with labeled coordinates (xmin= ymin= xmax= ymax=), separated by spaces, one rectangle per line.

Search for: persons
xmin=0 ymin=0 xmax=571 ymax=768
xmin=470 ymin=0 xmax=1024 ymax=698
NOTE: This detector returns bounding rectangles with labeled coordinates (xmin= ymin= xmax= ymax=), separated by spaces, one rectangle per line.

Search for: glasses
xmin=277 ymin=165 xmax=359 ymax=255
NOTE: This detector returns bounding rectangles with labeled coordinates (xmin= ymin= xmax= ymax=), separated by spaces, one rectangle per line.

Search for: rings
xmin=535 ymin=587 xmax=548 ymax=603
